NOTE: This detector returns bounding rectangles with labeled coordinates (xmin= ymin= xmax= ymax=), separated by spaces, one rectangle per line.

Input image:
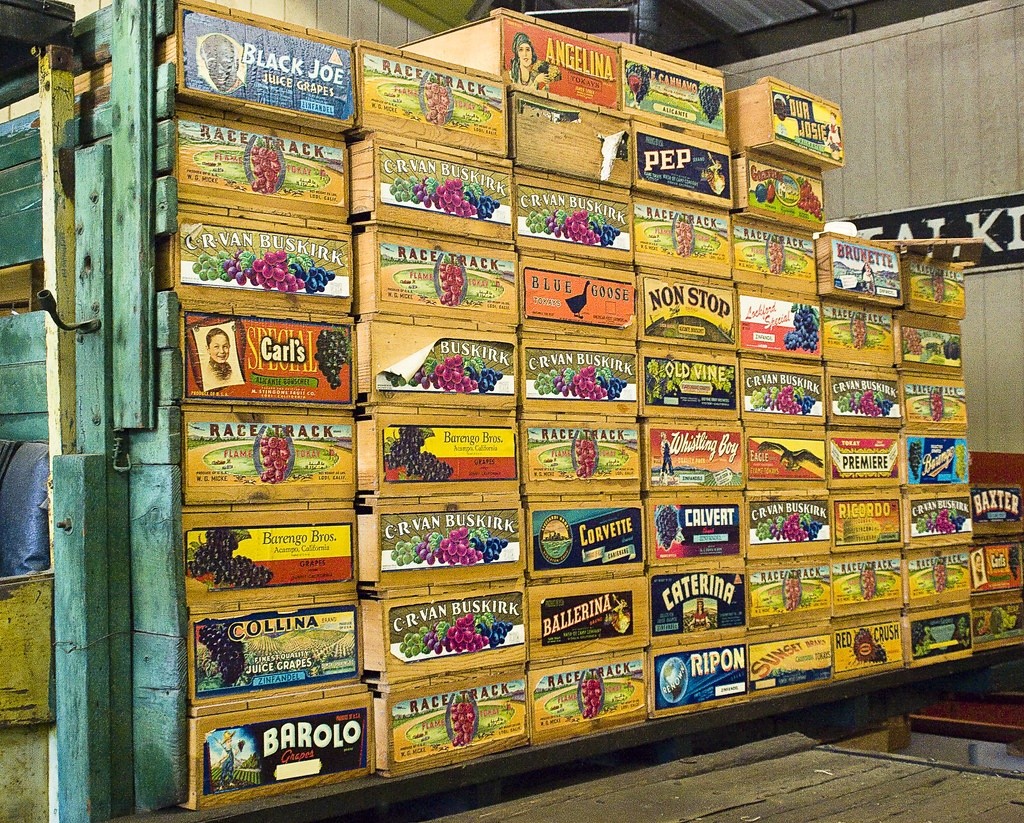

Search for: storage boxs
xmin=0 ymin=0 xmax=1024 ymax=823
